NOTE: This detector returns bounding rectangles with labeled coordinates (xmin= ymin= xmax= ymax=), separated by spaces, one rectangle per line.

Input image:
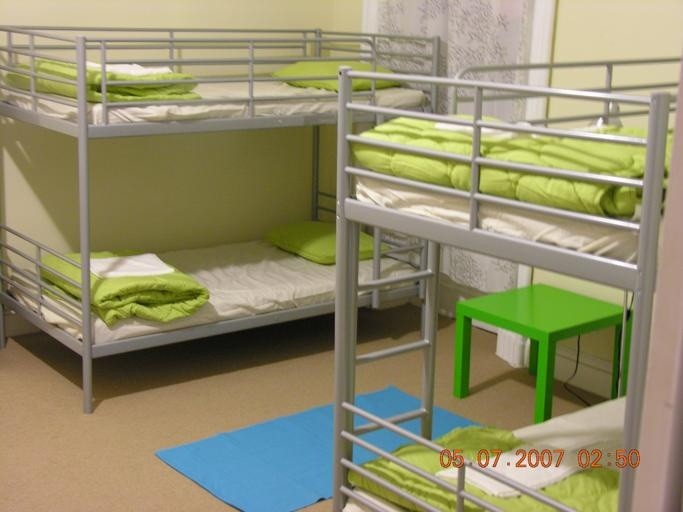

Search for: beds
xmin=0 ymin=28 xmax=440 ymax=415
xmin=332 ymin=57 xmax=683 ymax=512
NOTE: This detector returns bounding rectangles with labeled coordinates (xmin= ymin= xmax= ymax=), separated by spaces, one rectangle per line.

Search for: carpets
xmin=155 ymin=386 xmax=478 ymax=512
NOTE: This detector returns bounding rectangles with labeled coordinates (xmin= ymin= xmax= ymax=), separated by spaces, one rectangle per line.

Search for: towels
xmin=83 ymin=252 xmax=174 ymax=278
xmin=438 ymin=116 xmax=533 ymax=139
xmin=435 ymin=449 xmax=590 ymax=497
xmin=87 ymin=62 xmax=171 ymax=74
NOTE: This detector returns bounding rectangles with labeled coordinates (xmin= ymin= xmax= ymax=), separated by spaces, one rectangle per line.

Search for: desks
xmin=454 ymin=283 xmax=633 ymax=424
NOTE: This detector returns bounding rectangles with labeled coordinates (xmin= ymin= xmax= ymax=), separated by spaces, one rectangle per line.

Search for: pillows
xmin=264 ymin=221 xmax=389 ymax=265
xmin=273 ymin=60 xmax=399 ymax=90
